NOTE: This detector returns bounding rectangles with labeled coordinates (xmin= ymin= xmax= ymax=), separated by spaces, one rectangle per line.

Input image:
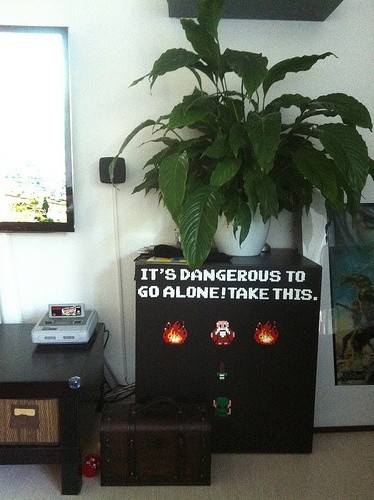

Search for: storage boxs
xmin=98 ymin=403 xmax=214 ymax=488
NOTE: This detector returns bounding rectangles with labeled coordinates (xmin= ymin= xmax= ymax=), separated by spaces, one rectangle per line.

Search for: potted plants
xmin=107 ymin=0 xmax=374 ymax=275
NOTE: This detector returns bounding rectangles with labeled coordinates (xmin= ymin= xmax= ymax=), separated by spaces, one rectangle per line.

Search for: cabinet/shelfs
xmin=133 ymin=246 xmax=322 ymax=456
xmin=0 ymin=321 xmax=109 ymax=496
